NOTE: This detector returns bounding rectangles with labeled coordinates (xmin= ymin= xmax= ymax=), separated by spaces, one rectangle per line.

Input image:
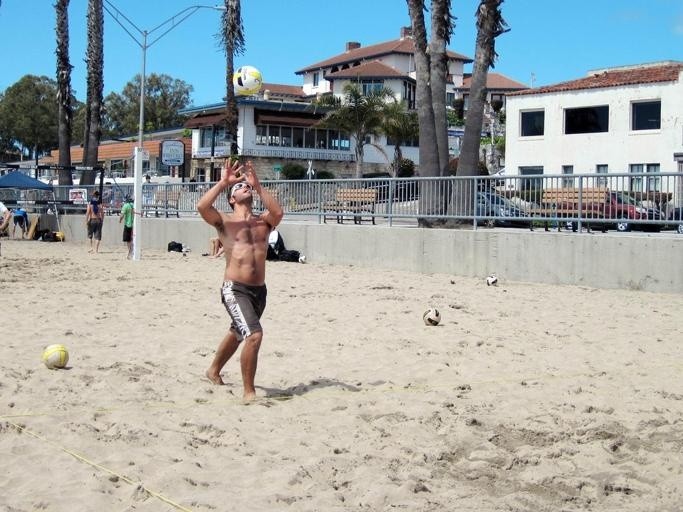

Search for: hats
xmin=492 ymin=167 xmax=505 ymax=186
xmin=475 ymin=190 xmax=530 ymax=229
xmin=666 ymin=206 xmax=683 ymax=234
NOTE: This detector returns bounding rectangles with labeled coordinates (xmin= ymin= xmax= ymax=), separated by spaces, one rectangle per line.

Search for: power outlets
xmin=346 ymin=175 xmax=418 ymax=208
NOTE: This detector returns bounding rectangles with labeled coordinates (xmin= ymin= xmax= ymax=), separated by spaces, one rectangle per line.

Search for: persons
xmin=84 ymin=194 xmax=104 ymax=253
xmin=0 ymin=199 xmax=12 ymax=231
xmin=119 ymin=194 xmax=133 ymax=260
xmin=12 ymin=209 xmax=28 ymax=239
xmin=210 ymin=236 xmax=220 ymax=256
xmin=143 ymin=175 xmax=154 ymax=217
xmin=197 ymin=156 xmax=284 ymax=406
xmin=210 ymin=246 xmax=224 ymax=259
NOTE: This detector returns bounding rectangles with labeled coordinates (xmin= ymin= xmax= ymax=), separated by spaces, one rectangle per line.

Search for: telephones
xmin=274 ymin=165 xmax=283 ymax=172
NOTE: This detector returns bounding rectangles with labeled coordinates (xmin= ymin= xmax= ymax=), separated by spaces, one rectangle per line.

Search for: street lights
xmin=278 ymin=250 xmax=299 ymax=262
xmin=168 ymin=241 xmax=182 ymax=252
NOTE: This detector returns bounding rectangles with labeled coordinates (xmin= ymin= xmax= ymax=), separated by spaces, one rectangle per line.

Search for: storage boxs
xmin=251 ymin=190 xmax=278 ymax=215
xmin=526 ymin=187 xmax=608 ymax=234
xmin=142 ymin=192 xmax=180 ymax=217
xmin=322 ymin=189 xmax=377 ymax=224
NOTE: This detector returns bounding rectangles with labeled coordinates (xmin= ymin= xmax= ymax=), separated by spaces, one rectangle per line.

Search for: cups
xmin=232 ymin=183 xmax=252 ymax=196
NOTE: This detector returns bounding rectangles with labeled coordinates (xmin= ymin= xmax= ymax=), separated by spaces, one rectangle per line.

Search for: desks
xmin=102 ymin=0 xmax=230 ymax=270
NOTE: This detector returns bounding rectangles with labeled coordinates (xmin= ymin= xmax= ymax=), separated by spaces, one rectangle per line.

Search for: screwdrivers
xmin=42 ymin=344 xmax=69 ymax=369
xmin=231 ymin=66 xmax=262 ymax=95
xmin=486 ymin=275 xmax=497 ymax=286
xmin=299 ymin=255 xmax=306 ymax=263
xmin=423 ymin=308 xmax=441 ymax=326
